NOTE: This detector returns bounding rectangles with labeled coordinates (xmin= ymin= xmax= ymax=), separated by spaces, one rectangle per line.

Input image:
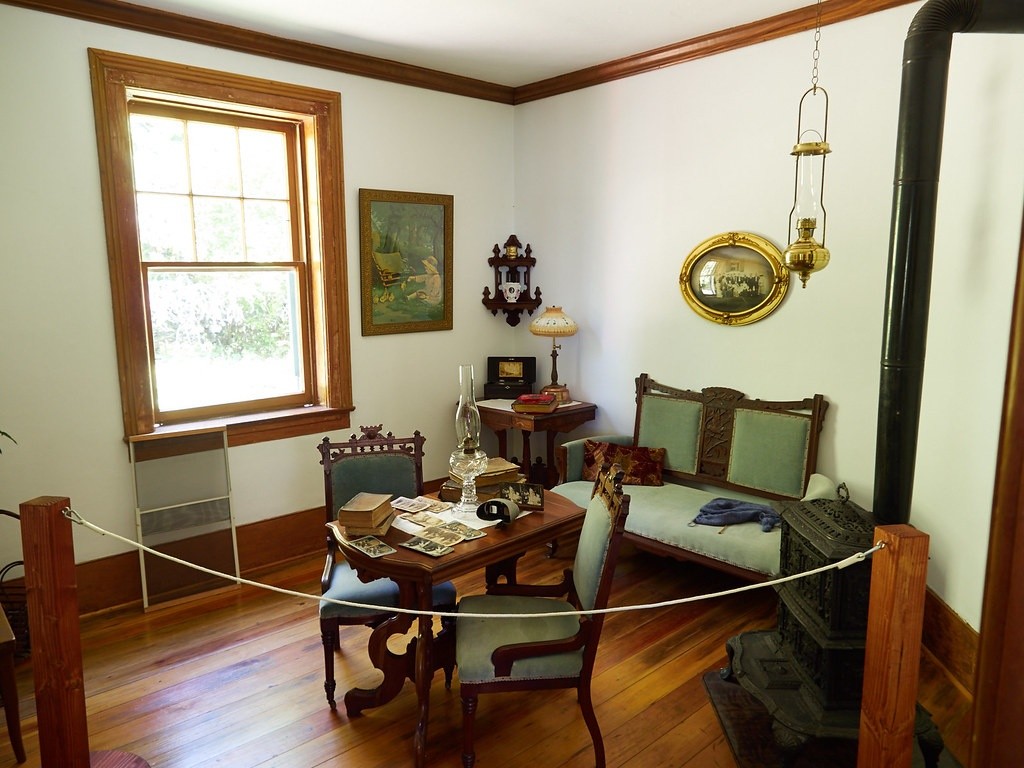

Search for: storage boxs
xmin=483 ymin=383 xmax=532 ymax=400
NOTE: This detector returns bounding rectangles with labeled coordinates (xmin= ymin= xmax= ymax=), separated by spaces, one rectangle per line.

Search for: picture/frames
xmin=499 ymin=482 xmax=545 ymax=511
xmin=358 ymin=188 xmax=454 ymax=336
xmin=679 ymin=231 xmax=790 ymax=327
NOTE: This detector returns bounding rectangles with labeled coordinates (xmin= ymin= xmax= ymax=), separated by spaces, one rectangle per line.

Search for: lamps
xmin=448 ymin=364 xmax=489 ymax=514
xmin=780 ymin=0 xmax=831 ymax=289
xmin=528 ymin=306 xmax=579 ymax=404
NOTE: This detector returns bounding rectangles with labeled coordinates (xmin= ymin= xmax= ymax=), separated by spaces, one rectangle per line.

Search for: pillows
xmin=581 ymin=438 xmax=668 ymax=487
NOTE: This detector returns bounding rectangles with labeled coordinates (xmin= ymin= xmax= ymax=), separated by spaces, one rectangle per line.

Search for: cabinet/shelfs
xmin=481 ymin=235 xmax=543 ymax=327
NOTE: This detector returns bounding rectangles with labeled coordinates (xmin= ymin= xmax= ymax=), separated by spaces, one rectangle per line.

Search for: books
xmin=512 ymin=393 xmax=558 ymax=414
xmin=440 ymin=457 xmax=527 ymax=501
xmin=338 ymin=492 xmax=396 ymax=536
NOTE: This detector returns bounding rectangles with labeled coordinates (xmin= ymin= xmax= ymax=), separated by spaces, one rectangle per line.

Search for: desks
xmin=456 ymin=396 xmax=599 ymax=489
xmin=0 ymin=603 xmax=28 ymax=763
xmin=325 ymin=489 xmax=587 ymax=768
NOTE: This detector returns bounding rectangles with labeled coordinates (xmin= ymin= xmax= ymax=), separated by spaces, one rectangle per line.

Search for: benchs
xmin=544 ymin=372 xmax=828 ymax=621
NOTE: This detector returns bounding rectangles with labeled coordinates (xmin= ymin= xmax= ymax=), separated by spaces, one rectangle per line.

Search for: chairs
xmin=317 ymin=423 xmax=458 ymax=712
xmin=452 ymin=461 xmax=632 ymax=767
